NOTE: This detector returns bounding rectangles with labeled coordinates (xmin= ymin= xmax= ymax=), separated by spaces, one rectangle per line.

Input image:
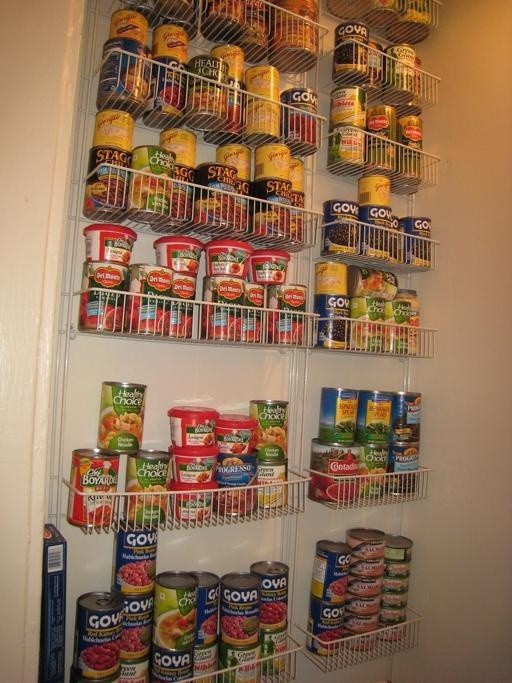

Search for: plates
xmin=47 ymin=0 xmax=449 ymax=683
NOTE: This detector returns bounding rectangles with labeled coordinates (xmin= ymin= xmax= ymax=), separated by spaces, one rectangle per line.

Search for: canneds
xmin=308 ymin=387 xmax=422 ymax=505
xmin=306 ymin=527 xmax=414 ymax=656
xmin=67 ymin=381 xmax=289 ymax=683
xmin=80 ymin=0 xmax=432 ymax=355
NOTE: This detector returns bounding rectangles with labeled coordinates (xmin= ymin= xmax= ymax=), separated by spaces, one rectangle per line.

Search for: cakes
xmin=308 ymin=387 xmax=422 ymax=505
xmin=306 ymin=527 xmax=414 ymax=656
